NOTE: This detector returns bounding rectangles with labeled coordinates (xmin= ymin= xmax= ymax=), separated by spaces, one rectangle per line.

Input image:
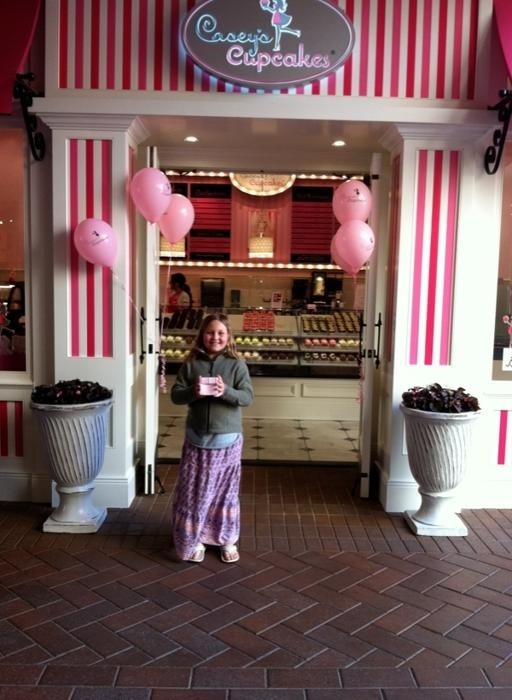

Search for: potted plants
xmin=31 ymin=377 xmax=117 ymax=533
xmin=397 ymin=381 xmax=482 ymax=542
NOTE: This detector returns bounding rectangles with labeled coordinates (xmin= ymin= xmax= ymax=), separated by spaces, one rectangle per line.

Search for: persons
xmin=171 ymin=314 xmax=254 ymax=563
xmin=165 ymin=273 xmax=192 ymax=313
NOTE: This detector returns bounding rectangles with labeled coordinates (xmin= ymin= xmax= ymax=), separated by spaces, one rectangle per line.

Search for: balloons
xmin=73 ymin=218 xmax=118 ymax=268
xmin=330 ymin=179 xmax=375 ymax=278
xmin=130 ymin=168 xmax=195 ymax=245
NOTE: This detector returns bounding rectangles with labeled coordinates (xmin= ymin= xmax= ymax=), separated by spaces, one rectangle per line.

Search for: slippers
xmin=221 ymin=543 xmax=239 ymax=563
xmin=187 ymin=543 xmax=205 ymax=562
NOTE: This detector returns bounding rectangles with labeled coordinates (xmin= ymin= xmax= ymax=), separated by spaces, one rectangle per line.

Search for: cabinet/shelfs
xmin=157 ymin=304 xmax=364 ymax=378
